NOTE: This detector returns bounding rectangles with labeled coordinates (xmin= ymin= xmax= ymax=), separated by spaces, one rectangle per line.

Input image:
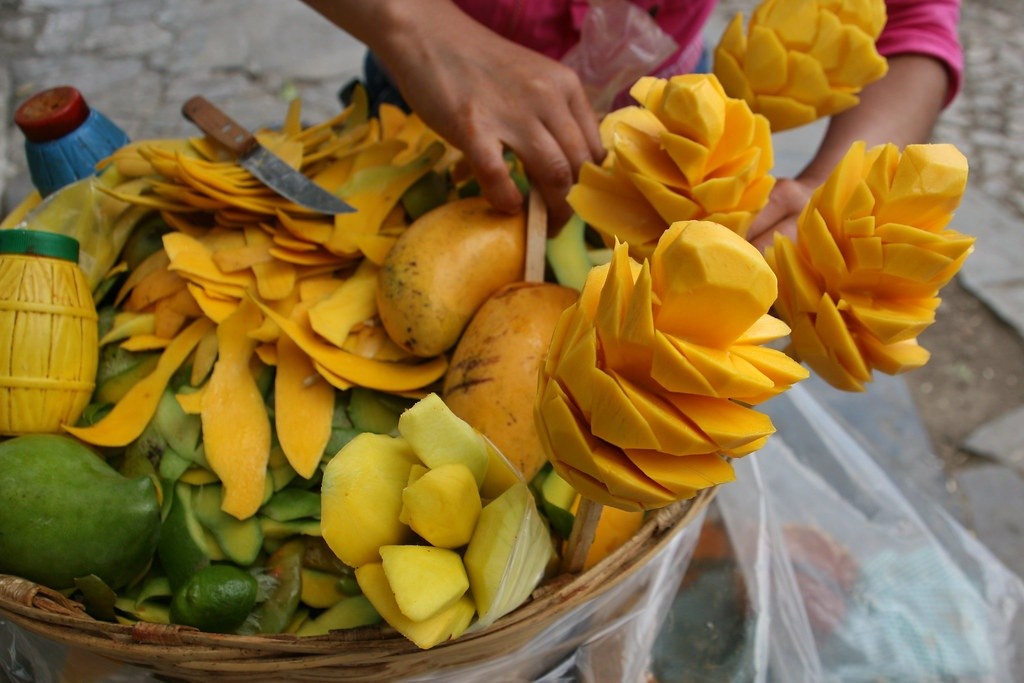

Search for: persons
xmin=294 ymin=0 xmax=966 ymax=261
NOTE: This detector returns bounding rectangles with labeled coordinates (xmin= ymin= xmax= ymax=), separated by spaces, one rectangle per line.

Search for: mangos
xmin=319 ymin=0 xmax=976 ymax=650
xmin=0 ymin=433 xmax=163 ymax=589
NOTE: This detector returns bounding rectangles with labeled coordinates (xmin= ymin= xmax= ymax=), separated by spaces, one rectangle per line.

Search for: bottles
xmin=13 ymin=86 xmax=133 ymax=200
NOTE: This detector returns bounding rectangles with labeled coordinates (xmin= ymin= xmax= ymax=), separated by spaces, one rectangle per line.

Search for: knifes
xmin=182 ymin=97 xmax=357 ymax=214
